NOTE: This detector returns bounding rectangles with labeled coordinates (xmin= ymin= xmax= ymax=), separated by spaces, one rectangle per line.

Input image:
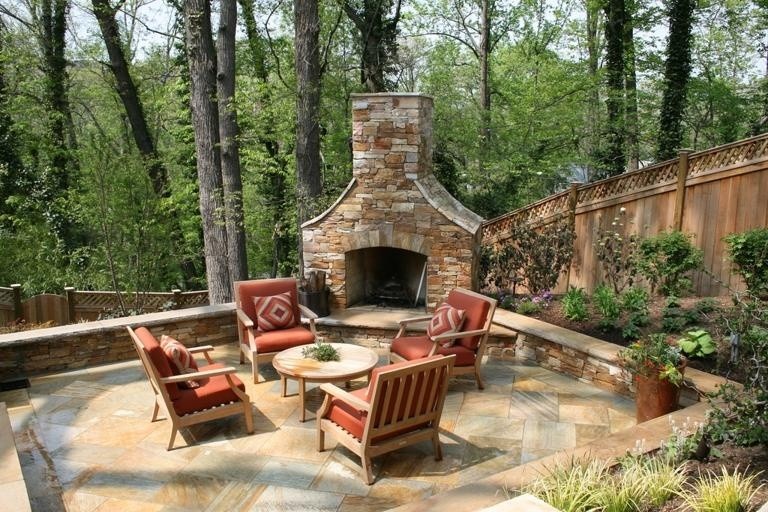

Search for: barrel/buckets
xmin=297 ymin=284 xmax=330 ymax=318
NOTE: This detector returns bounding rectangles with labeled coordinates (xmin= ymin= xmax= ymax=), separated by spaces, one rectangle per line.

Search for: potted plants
xmin=616 ymin=332 xmax=687 ymax=426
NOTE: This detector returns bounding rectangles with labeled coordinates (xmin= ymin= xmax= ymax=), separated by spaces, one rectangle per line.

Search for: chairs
xmin=232 ymin=275 xmax=320 ymax=385
xmin=386 ymin=285 xmax=498 ymax=395
xmin=123 ymin=319 xmax=256 ymax=453
xmin=314 ymin=352 xmax=462 ymax=488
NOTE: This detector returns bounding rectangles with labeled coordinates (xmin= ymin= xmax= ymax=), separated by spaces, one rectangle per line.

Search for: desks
xmin=271 ymin=341 xmax=381 ymax=421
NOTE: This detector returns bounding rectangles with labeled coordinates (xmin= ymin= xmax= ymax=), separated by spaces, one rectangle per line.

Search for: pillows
xmin=157 ymin=332 xmax=202 ymax=388
xmin=245 ymin=289 xmax=300 ymax=333
xmin=425 ymin=300 xmax=467 ymax=348
